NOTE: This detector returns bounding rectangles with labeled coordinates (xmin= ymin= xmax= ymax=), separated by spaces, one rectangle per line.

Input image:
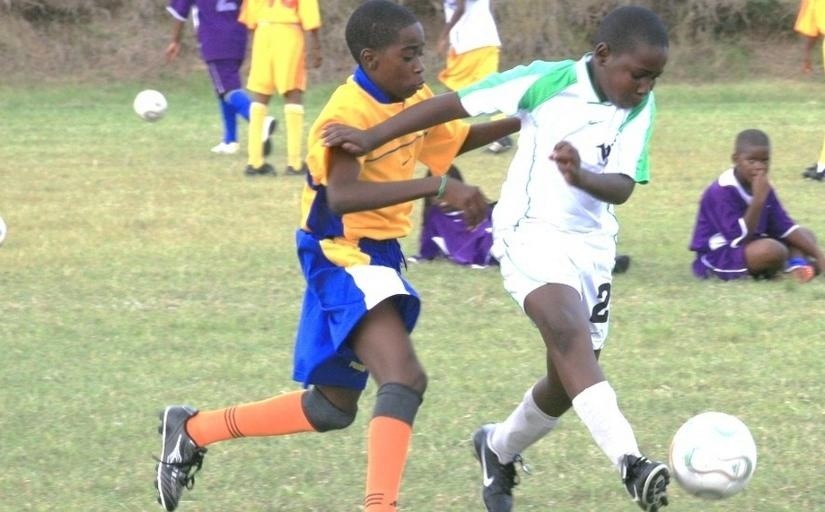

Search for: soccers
xmin=132 ymin=89 xmax=167 ymax=120
xmin=672 ymin=411 xmax=756 ymax=503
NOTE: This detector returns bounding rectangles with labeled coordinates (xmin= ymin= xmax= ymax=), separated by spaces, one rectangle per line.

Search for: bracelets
xmin=436 ymin=174 xmax=448 ymax=201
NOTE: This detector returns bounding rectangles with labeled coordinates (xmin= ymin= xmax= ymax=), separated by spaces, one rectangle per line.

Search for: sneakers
xmin=244 ymin=164 xmax=278 ymax=176
xmin=771 ymin=265 xmax=816 ymax=283
xmin=800 ymin=163 xmax=824 ymax=179
xmin=470 ymin=422 xmax=531 ymax=511
xmin=621 ymin=453 xmax=672 ymax=511
xmin=482 ymin=136 xmax=513 ymax=154
xmin=285 ymin=162 xmax=309 ymax=176
xmin=262 ymin=116 xmax=276 ymax=154
xmin=155 ymin=404 xmax=207 ymax=512
xmin=210 ymin=142 xmax=242 ymax=155
xmin=615 ymin=256 xmax=630 ymax=272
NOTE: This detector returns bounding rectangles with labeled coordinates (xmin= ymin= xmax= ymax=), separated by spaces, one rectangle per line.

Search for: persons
xmin=153 ymin=0 xmax=522 ymax=512
xmin=426 ymin=1 xmax=513 ymax=152
xmin=689 ymin=129 xmax=825 ymax=284
xmin=237 ymin=0 xmax=323 ymax=175
xmin=161 ymin=0 xmax=273 ymax=154
xmin=324 ymin=8 xmax=670 ymax=512
xmin=792 ymin=1 xmax=825 ymax=74
